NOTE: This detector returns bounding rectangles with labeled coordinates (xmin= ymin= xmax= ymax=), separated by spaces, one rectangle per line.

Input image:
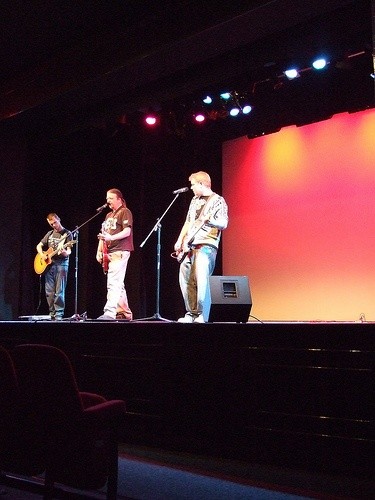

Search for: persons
xmin=36 ymin=213 xmax=73 ymax=321
xmin=173 ymin=170 xmax=229 ymax=323
xmin=97 ymin=189 xmax=134 ymax=320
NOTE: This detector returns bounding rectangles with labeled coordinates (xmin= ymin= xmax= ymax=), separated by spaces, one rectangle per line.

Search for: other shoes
xmin=97 ymin=314 xmax=114 ymax=320
xmin=54 ymin=316 xmax=62 ymax=320
xmin=116 ymin=313 xmax=126 ymax=319
xmin=194 ymin=315 xmax=205 ymax=323
xmin=178 ymin=315 xmax=194 ymax=322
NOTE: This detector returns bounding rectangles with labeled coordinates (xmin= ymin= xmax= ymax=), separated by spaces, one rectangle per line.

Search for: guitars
xmin=176 ymin=202 xmax=222 ymax=264
xmin=102 ymin=220 xmax=111 ymax=275
xmin=33 ymin=240 xmax=78 ymax=275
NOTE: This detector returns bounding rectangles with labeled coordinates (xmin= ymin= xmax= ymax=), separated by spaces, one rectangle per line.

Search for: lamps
xmin=185 ymin=91 xmax=255 ymax=127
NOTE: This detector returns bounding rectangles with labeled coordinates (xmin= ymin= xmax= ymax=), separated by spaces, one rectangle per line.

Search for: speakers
xmin=201 ymin=275 xmax=252 ymax=323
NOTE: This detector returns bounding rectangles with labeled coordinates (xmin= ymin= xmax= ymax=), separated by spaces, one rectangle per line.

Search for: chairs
xmin=0 ymin=345 xmax=127 ymax=499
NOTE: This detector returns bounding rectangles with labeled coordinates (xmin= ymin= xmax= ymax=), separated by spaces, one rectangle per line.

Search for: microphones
xmin=96 ymin=203 xmax=109 ymax=212
xmin=172 ymin=187 xmax=190 ymax=195
